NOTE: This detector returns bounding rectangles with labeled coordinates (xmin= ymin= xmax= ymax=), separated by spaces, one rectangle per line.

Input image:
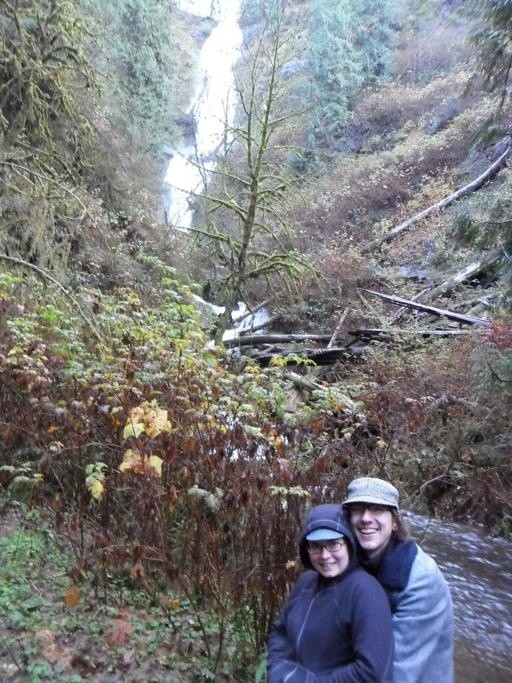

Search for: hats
xmin=341 ymin=477 xmax=399 ymax=508
xmin=307 ymin=527 xmax=344 ymax=542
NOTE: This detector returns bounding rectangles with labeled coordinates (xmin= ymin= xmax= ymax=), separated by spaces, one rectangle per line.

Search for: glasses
xmin=307 ymin=541 xmax=347 ymax=554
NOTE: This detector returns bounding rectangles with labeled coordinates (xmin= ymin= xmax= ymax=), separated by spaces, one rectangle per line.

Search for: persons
xmin=267 ymin=501 xmax=395 ymax=682
xmin=344 ymin=474 xmax=455 ymax=682
